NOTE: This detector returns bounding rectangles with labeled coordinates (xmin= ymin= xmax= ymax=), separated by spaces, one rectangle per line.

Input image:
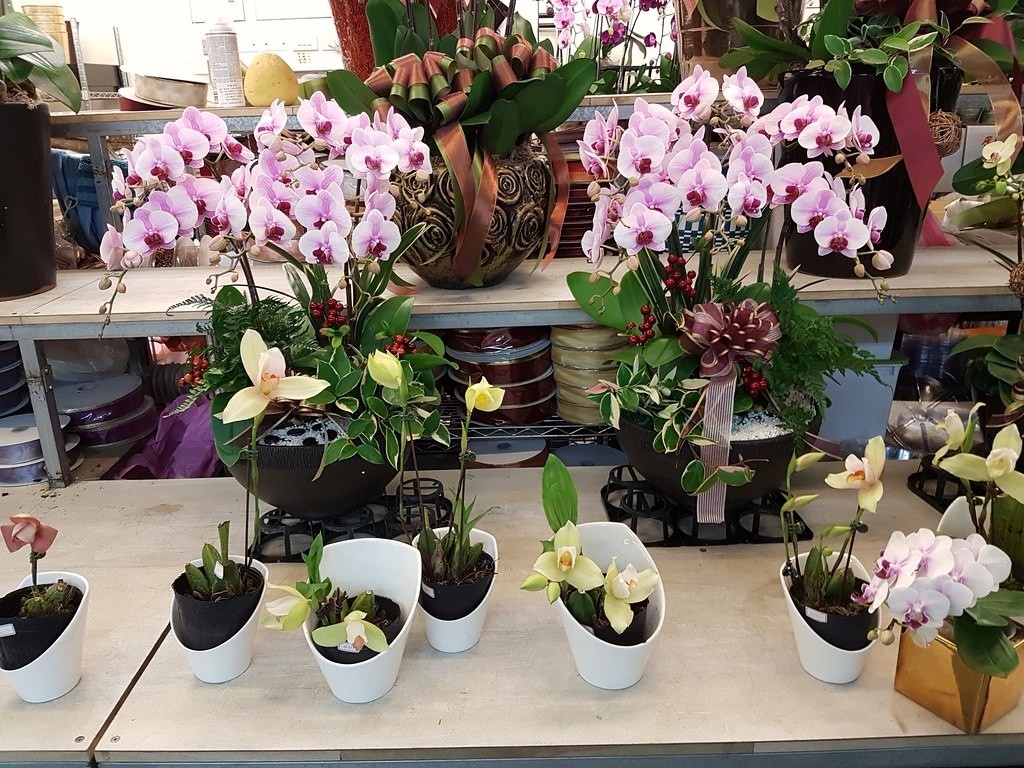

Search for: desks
xmin=51 ymin=85 xmax=1024 ymax=234
xmin=0 ymin=245 xmax=1024 ymax=488
xmin=0 ymin=459 xmax=1023 ymax=767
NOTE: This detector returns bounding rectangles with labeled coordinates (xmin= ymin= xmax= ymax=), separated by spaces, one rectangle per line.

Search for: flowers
xmin=0 ymin=0 xmax=1023 ymax=676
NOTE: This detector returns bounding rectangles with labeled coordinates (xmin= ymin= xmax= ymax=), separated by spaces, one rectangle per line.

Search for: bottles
xmin=203 ymin=18 xmax=245 ymax=108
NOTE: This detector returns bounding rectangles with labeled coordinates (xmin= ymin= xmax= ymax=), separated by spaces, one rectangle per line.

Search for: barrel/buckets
xmin=21 ymin=5 xmax=70 ymax=65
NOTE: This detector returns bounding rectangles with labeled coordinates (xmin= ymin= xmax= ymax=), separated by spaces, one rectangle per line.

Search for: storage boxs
xmin=815 ymin=349 xmax=910 ymax=444
xmin=829 ymin=315 xmax=901 ymax=360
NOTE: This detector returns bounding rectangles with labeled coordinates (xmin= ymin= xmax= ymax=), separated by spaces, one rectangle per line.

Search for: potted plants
xmin=0 ymin=11 xmax=82 ymax=301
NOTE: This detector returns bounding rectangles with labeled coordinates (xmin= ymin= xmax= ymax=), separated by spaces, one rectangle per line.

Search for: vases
xmin=411 ymin=526 xmax=499 ymax=653
xmin=0 ymin=583 xmax=83 ymax=671
xmin=0 ymin=571 xmax=90 ymax=703
xmin=423 ymin=552 xmax=494 ymax=621
xmin=773 ymin=68 xmax=964 ymax=280
xmin=781 ymin=551 xmax=881 ymax=684
xmin=554 ymin=142 xmax=619 ymax=257
xmin=169 ymin=555 xmax=269 ymax=684
xmin=330 ymin=0 xmax=459 ymax=82
xmin=302 ymin=537 xmax=423 ymax=704
xmin=549 ymin=523 xmax=666 ymax=690
xmin=171 ymin=564 xmax=264 ymax=650
xmin=316 ymin=595 xmax=401 ymax=665
xmin=675 ymin=1 xmax=804 ymax=90
xmin=449 ymin=327 xmax=557 ymax=427
xmin=570 ymin=573 xmax=650 ymax=646
xmin=550 ymin=324 xmax=633 ymax=427
xmin=388 ymin=143 xmax=552 ymax=290
xmin=790 ymin=573 xmax=878 ymax=652
xmin=228 ymin=432 xmax=404 ymax=519
